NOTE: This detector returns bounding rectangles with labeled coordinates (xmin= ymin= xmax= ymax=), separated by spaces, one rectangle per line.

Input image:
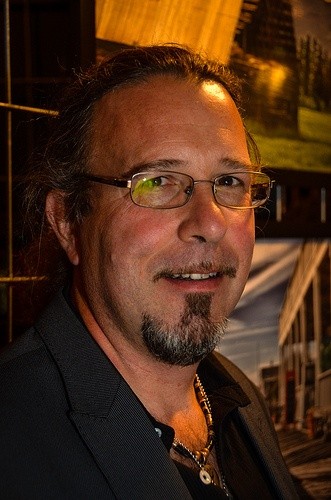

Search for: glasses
xmin=80 ymin=170 xmax=273 ymax=210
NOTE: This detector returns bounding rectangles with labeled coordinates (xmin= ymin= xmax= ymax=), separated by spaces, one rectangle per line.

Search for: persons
xmin=0 ymin=42 xmax=313 ymax=500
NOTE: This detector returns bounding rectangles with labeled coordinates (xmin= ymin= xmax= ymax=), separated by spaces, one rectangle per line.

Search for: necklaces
xmin=171 ymin=374 xmax=229 ymax=498
